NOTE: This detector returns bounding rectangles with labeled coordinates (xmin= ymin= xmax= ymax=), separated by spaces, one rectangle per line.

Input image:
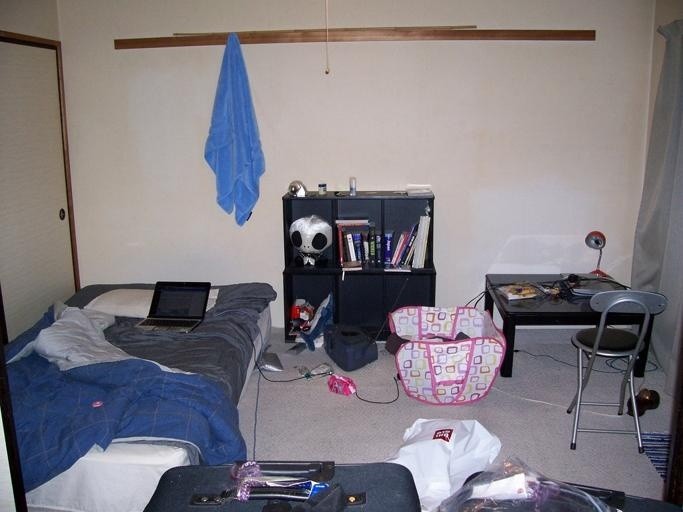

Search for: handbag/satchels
xmin=388 ymin=305 xmax=507 ymax=405
xmin=385 ymin=418 xmax=501 ymax=512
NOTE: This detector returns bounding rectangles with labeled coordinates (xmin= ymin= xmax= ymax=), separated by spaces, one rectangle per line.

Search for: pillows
xmin=84 ymin=289 xmax=219 ymax=319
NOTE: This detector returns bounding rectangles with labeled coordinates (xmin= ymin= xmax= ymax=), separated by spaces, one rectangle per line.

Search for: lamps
xmin=585 ymin=231 xmax=612 ymax=279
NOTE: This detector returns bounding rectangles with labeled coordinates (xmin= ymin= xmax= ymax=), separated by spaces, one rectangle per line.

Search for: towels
xmin=205 ymin=32 xmax=265 ymax=226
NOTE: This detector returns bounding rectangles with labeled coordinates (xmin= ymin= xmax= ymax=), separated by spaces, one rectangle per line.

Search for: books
xmin=406 ymin=187 xmax=432 ymax=197
xmin=335 ymin=213 xmax=429 ymax=273
xmin=497 ymin=285 xmax=537 ymax=301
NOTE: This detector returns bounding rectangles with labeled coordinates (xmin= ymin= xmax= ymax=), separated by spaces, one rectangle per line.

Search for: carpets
xmin=635 ymin=432 xmax=671 ymax=483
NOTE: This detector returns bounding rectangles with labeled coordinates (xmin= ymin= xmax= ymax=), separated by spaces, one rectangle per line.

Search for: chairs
xmin=568 ymin=290 xmax=668 ymax=453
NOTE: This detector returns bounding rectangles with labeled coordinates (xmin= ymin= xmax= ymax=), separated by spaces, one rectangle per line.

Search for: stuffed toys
xmin=289 ymin=215 xmax=332 ymax=267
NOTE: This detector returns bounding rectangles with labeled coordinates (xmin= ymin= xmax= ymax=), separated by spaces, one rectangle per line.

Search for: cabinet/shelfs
xmin=282 ymin=190 xmax=436 ymax=343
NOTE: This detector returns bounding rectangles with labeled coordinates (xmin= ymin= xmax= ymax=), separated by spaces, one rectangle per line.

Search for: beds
xmin=2 ymin=283 xmax=278 ymax=512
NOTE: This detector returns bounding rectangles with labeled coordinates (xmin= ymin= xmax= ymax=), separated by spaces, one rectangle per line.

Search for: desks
xmin=485 ymin=273 xmax=654 ymax=377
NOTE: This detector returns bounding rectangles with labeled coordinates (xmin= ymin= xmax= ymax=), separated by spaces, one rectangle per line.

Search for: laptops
xmin=134 ymin=281 xmax=211 ymax=334
